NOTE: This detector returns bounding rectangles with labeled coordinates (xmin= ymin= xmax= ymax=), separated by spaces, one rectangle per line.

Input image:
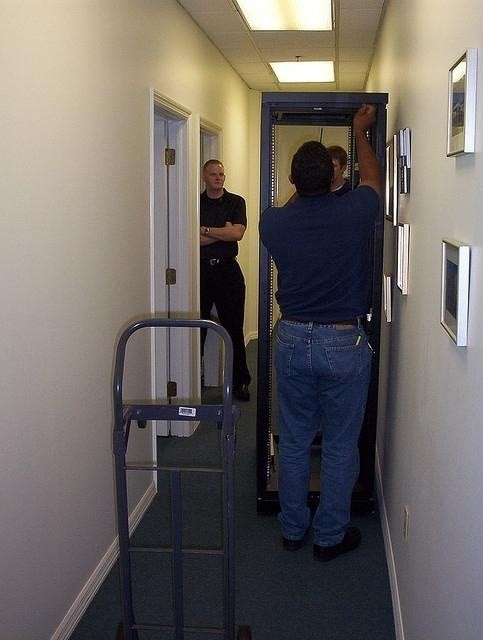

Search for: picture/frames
xmin=446 ymin=49 xmax=479 ymax=160
xmin=439 ymin=237 xmax=471 ymax=348
xmin=381 ymin=273 xmax=393 ymax=324
xmin=386 ymin=134 xmax=397 ymax=227
xmin=394 ymin=222 xmax=412 ymax=297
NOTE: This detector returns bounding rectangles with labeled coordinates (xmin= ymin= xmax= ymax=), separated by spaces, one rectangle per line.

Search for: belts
xmin=203 ymin=258 xmax=235 ymax=266
xmin=280 ymin=314 xmax=358 ymax=325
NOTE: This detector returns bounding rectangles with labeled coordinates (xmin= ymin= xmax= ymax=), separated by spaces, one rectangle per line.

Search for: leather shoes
xmin=233 ymin=384 xmax=250 ymax=401
xmin=282 ymin=526 xmax=361 ymax=562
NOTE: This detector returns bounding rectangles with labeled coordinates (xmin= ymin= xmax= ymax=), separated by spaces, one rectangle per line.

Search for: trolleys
xmin=113 ymin=315 xmax=252 ymax=639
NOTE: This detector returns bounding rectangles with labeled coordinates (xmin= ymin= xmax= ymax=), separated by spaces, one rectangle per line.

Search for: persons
xmin=328 ymin=145 xmax=352 ymax=195
xmin=258 ymin=102 xmax=382 ymax=564
xmin=200 ymin=159 xmax=252 ymax=401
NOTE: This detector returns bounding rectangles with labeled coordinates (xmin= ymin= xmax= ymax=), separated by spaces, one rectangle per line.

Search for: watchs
xmin=205 ymin=227 xmax=210 ymax=236
